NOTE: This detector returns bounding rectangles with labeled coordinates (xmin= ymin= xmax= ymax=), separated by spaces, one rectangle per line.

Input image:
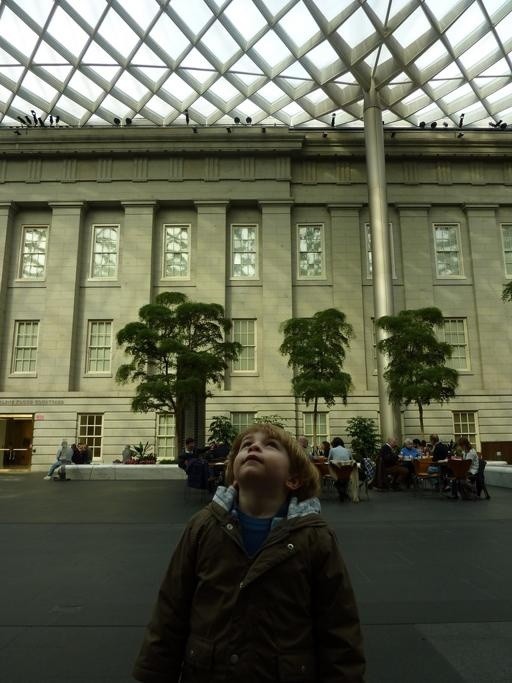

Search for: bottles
xmin=400 ymin=451 xmax=431 ymax=461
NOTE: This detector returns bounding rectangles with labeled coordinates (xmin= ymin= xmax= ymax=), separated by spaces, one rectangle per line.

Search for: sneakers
xmin=43 ymin=475 xmax=52 ymax=481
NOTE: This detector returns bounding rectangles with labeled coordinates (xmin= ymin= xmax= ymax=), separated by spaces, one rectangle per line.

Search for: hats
xmin=112 ymin=459 xmax=122 ymax=463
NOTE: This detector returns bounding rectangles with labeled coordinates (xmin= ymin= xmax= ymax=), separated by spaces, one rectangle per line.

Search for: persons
xmin=178 ymin=432 xmax=481 ymax=501
xmin=46 ymin=441 xmax=137 ymax=481
xmin=127 ymin=419 xmax=368 ymax=683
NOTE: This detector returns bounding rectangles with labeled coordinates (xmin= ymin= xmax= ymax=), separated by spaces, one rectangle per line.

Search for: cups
xmin=318 ymin=456 xmax=324 ymax=463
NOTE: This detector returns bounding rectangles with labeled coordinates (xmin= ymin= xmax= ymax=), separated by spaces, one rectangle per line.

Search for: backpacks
xmin=184 ymin=456 xmax=209 ymax=491
